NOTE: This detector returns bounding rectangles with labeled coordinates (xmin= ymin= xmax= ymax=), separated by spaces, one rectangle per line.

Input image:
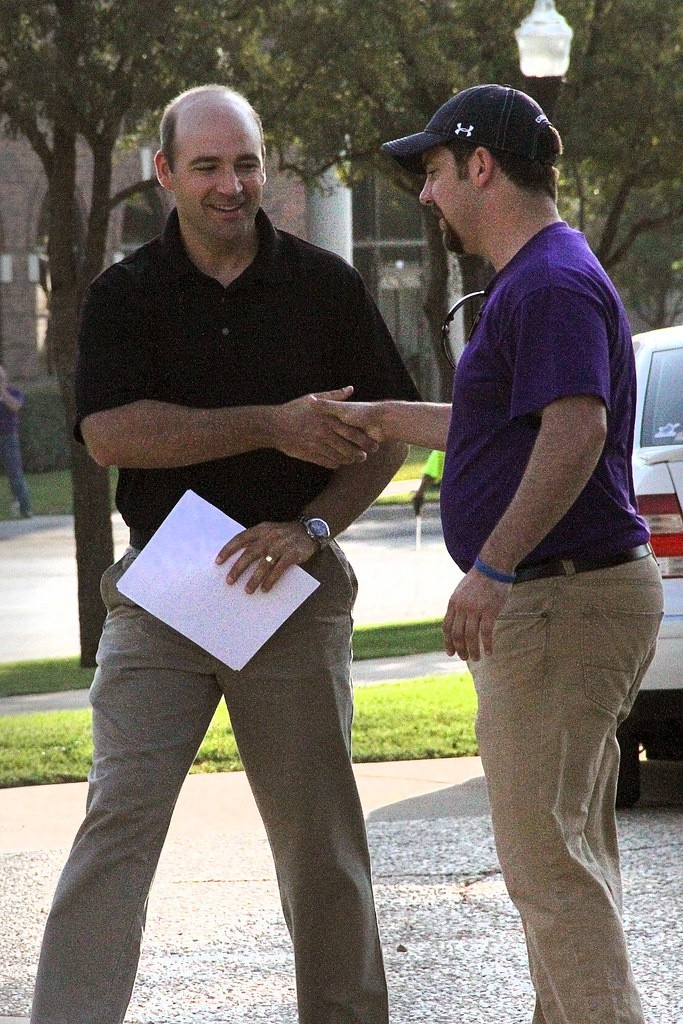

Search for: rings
xmin=265 ymin=554 xmax=275 ymax=565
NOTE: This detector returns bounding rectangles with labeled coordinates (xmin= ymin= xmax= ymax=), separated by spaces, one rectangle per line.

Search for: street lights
xmin=513 ymin=0 xmax=574 ymax=130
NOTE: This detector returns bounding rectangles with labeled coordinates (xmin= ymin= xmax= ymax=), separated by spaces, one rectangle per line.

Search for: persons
xmin=30 ymin=84 xmax=424 ymax=1024
xmin=307 ymin=84 xmax=665 ymax=1024
xmin=0 ymin=364 xmax=32 ymax=518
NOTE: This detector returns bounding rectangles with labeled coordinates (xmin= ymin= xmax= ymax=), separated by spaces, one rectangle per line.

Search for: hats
xmin=380 ymin=85 xmax=562 ymax=176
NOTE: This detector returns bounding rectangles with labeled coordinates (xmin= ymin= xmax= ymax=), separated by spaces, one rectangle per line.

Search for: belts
xmin=516 ymin=539 xmax=651 ymax=583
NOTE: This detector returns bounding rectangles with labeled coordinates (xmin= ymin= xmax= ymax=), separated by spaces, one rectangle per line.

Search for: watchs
xmin=297 ymin=513 xmax=330 ymax=549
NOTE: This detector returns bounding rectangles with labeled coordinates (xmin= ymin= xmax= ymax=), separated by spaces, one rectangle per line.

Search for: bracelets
xmin=475 ymin=556 xmax=515 ymax=583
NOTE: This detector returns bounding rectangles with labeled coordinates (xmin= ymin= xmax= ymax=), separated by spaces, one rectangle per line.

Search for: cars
xmin=617 ymin=323 xmax=683 ymax=813
